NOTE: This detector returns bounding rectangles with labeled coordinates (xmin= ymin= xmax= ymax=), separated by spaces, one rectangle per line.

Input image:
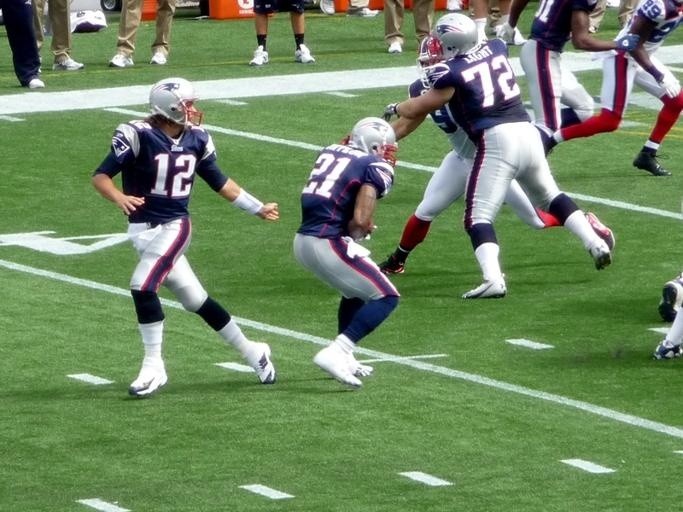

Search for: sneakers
xmin=633 ymin=150 xmax=673 ymax=176
xmin=51 ymin=55 xmax=167 ymax=72
xmin=656 ymin=276 xmax=683 ymax=322
xmin=248 ymin=45 xmax=269 ymax=66
xmin=319 ymin=0 xmax=402 ymax=53
xmin=378 ymin=253 xmax=405 ymax=275
xmin=474 ymin=17 xmax=527 ymax=44
xmin=584 ymin=211 xmax=616 ymax=251
xmin=27 ymin=78 xmax=45 ymax=88
xmin=446 ymin=0 xmax=463 ymax=11
xmin=588 ymin=239 xmax=611 ymax=270
xmin=588 ymin=24 xmax=599 ymax=34
xmin=127 ymin=364 xmax=168 ymax=397
xmin=295 ymin=43 xmax=316 ymax=64
xmin=247 ymin=342 xmax=372 ymax=388
xmin=462 ymin=272 xmax=507 ymax=299
xmin=652 ymin=339 xmax=683 ymax=360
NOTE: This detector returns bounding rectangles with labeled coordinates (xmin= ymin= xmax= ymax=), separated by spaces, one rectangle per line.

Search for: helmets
xmin=427 ymin=13 xmax=482 ymax=66
xmin=348 ymin=116 xmax=399 ymax=168
xmin=149 ymin=77 xmax=202 ymax=131
xmin=417 ymin=38 xmax=445 ymax=88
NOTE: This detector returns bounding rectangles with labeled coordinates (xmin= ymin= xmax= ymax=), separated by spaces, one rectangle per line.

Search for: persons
xmin=485 ymin=1 xmax=530 ymax=45
xmin=342 ymin=35 xmax=618 ymax=273
xmin=545 ymin=1 xmax=682 ymax=176
xmin=655 ymin=273 xmax=682 ymax=362
xmin=383 ymin=1 xmax=432 ymax=52
xmin=109 ymin=0 xmax=176 ymax=67
xmin=505 ymin=1 xmax=640 ymax=152
xmin=89 ymin=74 xmax=280 ymax=399
xmin=384 ymin=12 xmax=611 ymax=298
xmin=1 ymin=2 xmax=83 ymax=89
xmin=292 ymin=114 xmax=397 ymax=386
xmin=249 ymin=0 xmax=315 ymax=65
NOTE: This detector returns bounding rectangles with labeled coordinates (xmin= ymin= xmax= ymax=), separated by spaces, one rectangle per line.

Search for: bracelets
xmin=231 ymin=187 xmax=264 ymax=216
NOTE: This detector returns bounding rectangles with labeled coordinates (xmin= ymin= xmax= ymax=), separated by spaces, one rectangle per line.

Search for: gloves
xmin=658 ymin=73 xmax=682 ymax=99
xmin=615 ymin=33 xmax=641 ymax=51
